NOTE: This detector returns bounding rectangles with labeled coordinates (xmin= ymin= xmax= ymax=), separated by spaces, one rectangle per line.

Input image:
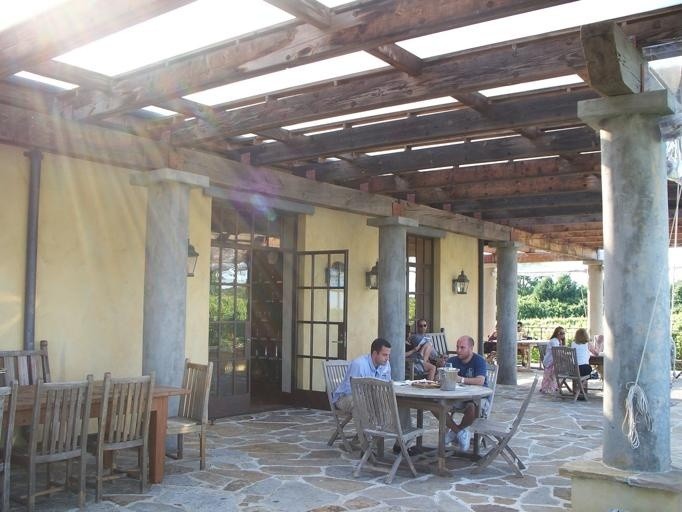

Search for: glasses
xmin=558 ymin=332 xmax=565 ymax=335
xmin=419 ymin=324 xmax=426 ymax=328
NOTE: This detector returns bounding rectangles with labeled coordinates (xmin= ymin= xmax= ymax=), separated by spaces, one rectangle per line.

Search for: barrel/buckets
xmin=437 ymin=363 xmax=460 ymax=391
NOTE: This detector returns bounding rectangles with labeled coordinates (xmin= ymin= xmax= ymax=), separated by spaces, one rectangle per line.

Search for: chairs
xmin=165 ymin=358 xmax=214 ymax=470
xmin=469 ymin=375 xmax=539 ymax=478
xmin=0 ymin=340 xmax=53 ymax=386
xmin=349 ymin=375 xmax=423 ymax=484
xmin=67 ymin=371 xmax=158 ymax=503
xmin=407 ymin=328 xmax=457 ymax=378
xmin=536 ymin=342 xmax=572 ymax=392
xmin=551 ymin=346 xmax=590 ymax=403
xmin=446 ymin=361 xmax=500 ymax=447
xmin=11 ymin=374 xmax=96 ymax=512
xmin=320 ymin=358 xmax=367 ymax=452
xmin=1 ymin=378 xmax=20 ymax=512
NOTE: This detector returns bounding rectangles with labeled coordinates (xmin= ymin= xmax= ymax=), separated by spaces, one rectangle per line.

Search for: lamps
xmin=365 ymin=262 xmax=380 ymax=290
xmin=188 ymin=238 xmax=199 ymax=278
xmin=453 ymin=269 xmax=470 ymax=294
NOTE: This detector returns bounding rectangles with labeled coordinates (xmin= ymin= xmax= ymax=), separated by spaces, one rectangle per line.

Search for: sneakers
xmin=445 ymin=430 xmax=456 ymax=445
xmin=456 ymin=430 xmax=471 ymax=453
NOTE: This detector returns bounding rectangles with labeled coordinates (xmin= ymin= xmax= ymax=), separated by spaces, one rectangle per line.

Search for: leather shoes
xmin=360 ymin=450 xmax=375 ymax=461
xmin=392 ymin=445 xmax=413 ymax=456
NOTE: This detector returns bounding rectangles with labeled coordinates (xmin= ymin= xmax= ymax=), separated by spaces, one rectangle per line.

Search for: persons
xmin=541 ymin=327 xmax=565 ymax=394
xmin=413 ymin=319 xmax=448 ymax=366
xmin=431 ymin=336 xmax=493 ymax=451
xmin=488 ymin=325 xmax=497 ymax=362
xmin=405 ymin=324 xmax=436 ymax=382
xmin=331 ymin=338 xmax=414 ymax=461
xmin=571 ymin=328 xmax=599 ymax=377
xmin=517 ymin=322 xmax=528 ymax=367
